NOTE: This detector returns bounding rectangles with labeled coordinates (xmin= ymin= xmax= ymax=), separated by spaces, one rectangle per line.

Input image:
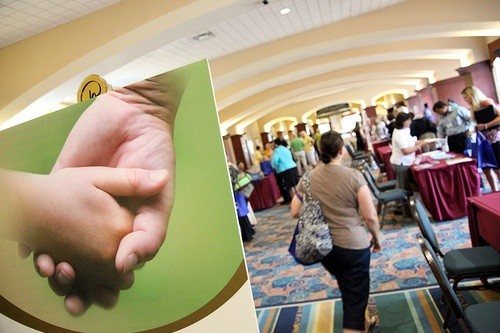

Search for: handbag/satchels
xmin=287 ymin=171 xmax=332 ymax=266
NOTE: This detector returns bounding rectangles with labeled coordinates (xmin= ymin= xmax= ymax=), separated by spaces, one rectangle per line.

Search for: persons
xmin=228 ymin=162 xmax=255 ymax=243
xmin=448 ymin=99 xmax=459 ymax=110
xmin=461 ymin=85 xmax=500 ymax=191
xmin=433 ymin=101 xmax=474 ymax=157
xmin=424 ymin=103 xmax=432 ymax=122
xmin=237 ymin=161 xmax=251 ymax=175
xmin=291 ymin=131 xmax=381 ymax=333
xmin=365 ymin=111 xmax=406 ymax=145
xmin=390 ymin=112 xmax=430 ymax=223
xmin=0 ymin=69 xmax=193 ymax=317
xmin=353 ymin=122 xmax=365 ymax=151
xmin=411 ymin=114 xmax=437 ymax=152
xmin=253 ymin=124 xmax=322 ymax=205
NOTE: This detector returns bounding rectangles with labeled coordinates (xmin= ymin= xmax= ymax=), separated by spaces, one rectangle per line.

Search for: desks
xmin=408 ymin=147 xmax=481 ymax=221
xmin=245 ymin=169 xmax=281 ymax=211
xmin=371 ymin=139 xmax=392 ymax=163
xmin=466 ymin=190 xmax=500 ymax=251
xmin=377 ymin=146 xmax=396 ymax=182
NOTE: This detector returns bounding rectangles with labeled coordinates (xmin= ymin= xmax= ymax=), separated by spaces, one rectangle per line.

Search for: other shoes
xmin=366 ymin=315 xmax=376 ymax=333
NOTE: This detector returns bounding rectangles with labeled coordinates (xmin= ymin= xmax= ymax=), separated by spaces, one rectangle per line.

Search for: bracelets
xmin=485 ymin=124 xmax=487 ymax=130
xmin=418 ymin=145 xmax=421 ymax=150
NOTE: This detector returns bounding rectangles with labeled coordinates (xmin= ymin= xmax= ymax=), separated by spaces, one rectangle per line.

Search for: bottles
xmin=435 ymin=140 xmax=442 ymax=151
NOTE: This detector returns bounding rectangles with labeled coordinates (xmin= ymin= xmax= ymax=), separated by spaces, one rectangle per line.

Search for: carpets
xmin=255 ymin=275 xmax=500 ymax=333
xmin=242 ymin=185 xmax=486 ymax=307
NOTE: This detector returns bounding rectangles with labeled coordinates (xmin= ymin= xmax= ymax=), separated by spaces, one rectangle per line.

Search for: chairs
xmin=346 ymin=141 xmax=373 ymax=170
xmin=361 ymin=170 xmax=416 ymax=230
xmin=409 ymin=195 xmax=500 ymax=330
xmin=362 ymin=163 xmax=403 ymax=210
xmin=416 ymin=236 xmax=500 ymax=333
